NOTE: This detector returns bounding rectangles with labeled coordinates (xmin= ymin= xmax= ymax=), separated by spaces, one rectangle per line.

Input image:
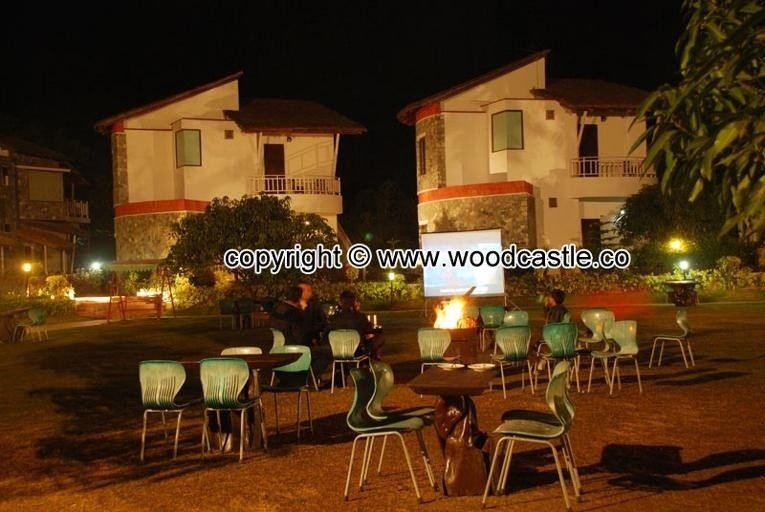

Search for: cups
xmin=367 ymin=314 xmax=376 ymax=329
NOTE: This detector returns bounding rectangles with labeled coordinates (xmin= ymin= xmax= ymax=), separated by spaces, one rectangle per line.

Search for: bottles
xmin=328 ymin=306 xmax=335 ymax=318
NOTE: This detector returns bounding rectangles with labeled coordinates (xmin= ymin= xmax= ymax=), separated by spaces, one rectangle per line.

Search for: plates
xmin=437 ymin=363 xmax=466 ymax=371
xmin=467 ymin=363 xmax=496 ymax=373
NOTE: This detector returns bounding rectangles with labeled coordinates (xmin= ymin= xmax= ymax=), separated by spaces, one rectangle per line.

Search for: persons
xmin=295 ymin=283 xmax=328 ymax=344
xmin=260 ymin=286 xmax=312 ymax=387
xmin=525 ymin=289 xmax=567 ymax=373
xmin=330 ymin=288 xmax=385 ymax=362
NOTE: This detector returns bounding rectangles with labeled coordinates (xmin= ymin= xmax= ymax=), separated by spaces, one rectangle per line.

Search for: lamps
xmin=678 ymin=260 xmax=691 ymax=280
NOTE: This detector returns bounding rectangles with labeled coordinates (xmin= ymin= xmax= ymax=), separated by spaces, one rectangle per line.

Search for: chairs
xmin=11 ymin=296 xmax=696 ymax=511
